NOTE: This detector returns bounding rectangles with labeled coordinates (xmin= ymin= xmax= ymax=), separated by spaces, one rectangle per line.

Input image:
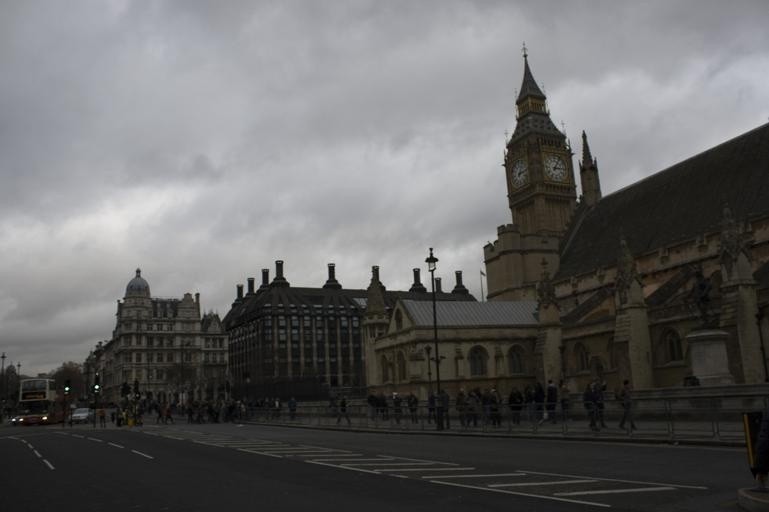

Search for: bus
xmin=18 ymin=377 xmax=72 ymax=426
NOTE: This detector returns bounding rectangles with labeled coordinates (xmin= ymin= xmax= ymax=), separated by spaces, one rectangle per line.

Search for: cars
xmin=11 ymin=415 xmax=23 ymax=426
xmin=68 ymin=407 xmax=95 ymax=424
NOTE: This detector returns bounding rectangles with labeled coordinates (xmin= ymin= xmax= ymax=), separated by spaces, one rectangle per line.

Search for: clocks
xmin=543 ymin=152 xmax=568 ymax=183
xmin=508 ymin=159 xmax=530 ymax=189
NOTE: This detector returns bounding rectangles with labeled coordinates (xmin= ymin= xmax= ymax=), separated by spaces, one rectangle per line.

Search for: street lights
xmin=425 ymin=248 xmax=446 ymax=431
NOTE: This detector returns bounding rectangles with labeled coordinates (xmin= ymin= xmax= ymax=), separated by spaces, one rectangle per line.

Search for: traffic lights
xmin=92 ymin=384 xmax=101 ymax=395
xmin=63 ymin=385 xmax=72 ymax=396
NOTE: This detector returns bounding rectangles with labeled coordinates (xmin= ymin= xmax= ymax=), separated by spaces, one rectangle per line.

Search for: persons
xmin=329 ymin=386 xmax=339 ymax=409
xmin=288 ymin=397 xmax=298 ymax=419
xmin=367 ymin=378 xmax=571 ymax=430
xmin=97 ymin=406 xmax=107 ymax=429
xmin=110 ymin=394 xmax=282 ymax=429
xmin=583 ymin=375 xmax=609 ymax=431
xmin=616 ymin=379 xmax=639 ymax=431
xmin=337 ymin=394 xmax=353 ymax=426
